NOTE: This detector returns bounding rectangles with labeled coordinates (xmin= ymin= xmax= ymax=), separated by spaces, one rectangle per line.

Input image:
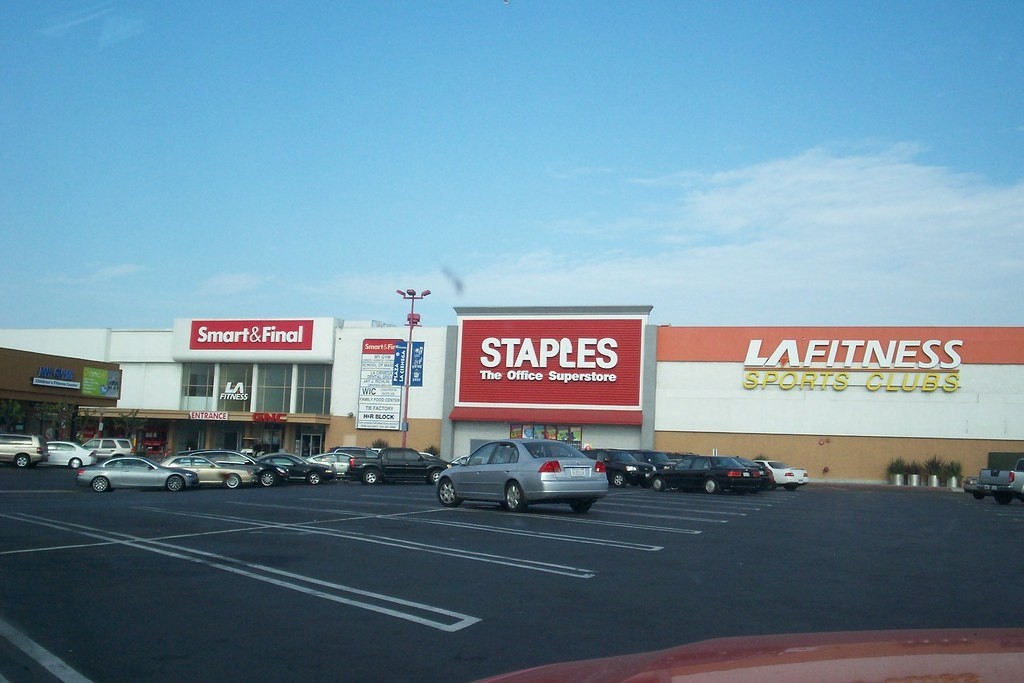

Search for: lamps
xmin=347 ymin=412 xmax=353 ymax=416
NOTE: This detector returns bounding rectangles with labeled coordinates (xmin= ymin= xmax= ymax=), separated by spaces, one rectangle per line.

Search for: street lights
xmin=397 ymin=288 xmax=432 ymax=447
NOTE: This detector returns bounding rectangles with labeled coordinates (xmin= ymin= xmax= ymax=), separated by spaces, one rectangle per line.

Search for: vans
xmin=80 ymin=438 xmax=135 ymax=465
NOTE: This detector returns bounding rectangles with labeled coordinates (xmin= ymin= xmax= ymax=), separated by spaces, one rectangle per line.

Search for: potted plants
xmin=886 ymin=454 xmax=964 ymax=488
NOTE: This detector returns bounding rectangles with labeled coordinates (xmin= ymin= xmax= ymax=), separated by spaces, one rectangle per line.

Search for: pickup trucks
xmin=345 ymin=447 xmax=452 ymax=487
xmin=963 ymin=457 xmax=1024 ymax=505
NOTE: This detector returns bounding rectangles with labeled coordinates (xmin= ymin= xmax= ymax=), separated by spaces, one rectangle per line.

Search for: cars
xmin=178 ymin=449 xmax=292 ymax=488
xmin=34 ymin=441 xmax=97 ymax=470
xmin=159 ymin=454 xmax=254 ymax=489
xmin=255 ymin=452 xmax=336 ymax=488
xmin=434 ymin=438 xmax=610 ymax=514
xmin=576 ymin=448 xmax=811 ymax=497
xmin=74 ymin=455 xmax=199 ymax=492
xmin=303 ymin=445 xmax=487 ymax=489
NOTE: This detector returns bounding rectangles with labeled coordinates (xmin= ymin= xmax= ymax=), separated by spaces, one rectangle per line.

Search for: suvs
xmin=0 ymin=433 xmax=49 ymax=469
